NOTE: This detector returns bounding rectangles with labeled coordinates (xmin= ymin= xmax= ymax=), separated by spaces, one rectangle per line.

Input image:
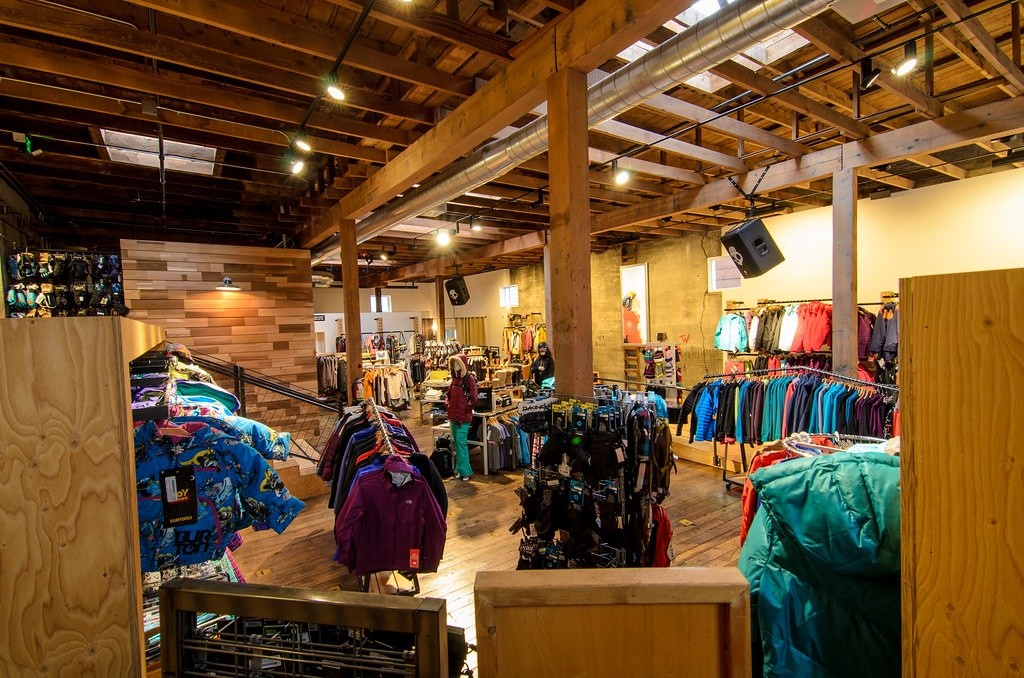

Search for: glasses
xmin=539 ymin=347 xmax=547 ymax=352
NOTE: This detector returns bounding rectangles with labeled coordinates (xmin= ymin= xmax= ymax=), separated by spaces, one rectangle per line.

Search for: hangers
xmin=317 ymin=312 xmax=545 ymax=375
xmin=156 ymin=355 xmax=194 ymax=439
xmin=704 ymin=291 xmax=900 ymax=398
xmin=361 ymin=398 xmax=395 ymax=458
xmin=780 ymin=430 xmax=887 ymax=459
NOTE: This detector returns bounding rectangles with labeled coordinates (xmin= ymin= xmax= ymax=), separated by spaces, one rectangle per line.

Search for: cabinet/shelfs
xmin=621 ymin=344 xmax=642 ymax=393
xmin=643 ymin=342 xmax=688 ymax=424
xmin=485 ymin=388 xmax=658 ymax=570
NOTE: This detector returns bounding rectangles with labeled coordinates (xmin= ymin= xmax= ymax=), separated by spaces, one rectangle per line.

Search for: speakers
xmin=721 ymin=218 xmax=785 ymax=279
xmin=444 ymin=277 xmax=470 ymax=306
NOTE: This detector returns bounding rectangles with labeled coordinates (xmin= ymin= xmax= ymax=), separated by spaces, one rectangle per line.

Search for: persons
xmin=529 ymin=341 xmax=557 ymax=387
xmin=444 ymin=353 xmax=479 ymax=480
xmin=621 ymin=289 xmax=642 ymax=344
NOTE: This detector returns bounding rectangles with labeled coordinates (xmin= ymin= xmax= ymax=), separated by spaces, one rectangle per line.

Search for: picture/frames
xmin=619 ymin=262 xmax=651 ymax=348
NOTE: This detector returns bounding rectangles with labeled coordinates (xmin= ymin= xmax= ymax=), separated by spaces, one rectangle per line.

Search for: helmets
xmin=538 ymin=342 xmax=548 ymax=352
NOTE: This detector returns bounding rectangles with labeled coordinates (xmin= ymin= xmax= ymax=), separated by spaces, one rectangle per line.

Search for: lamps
xmin=215 ymin=277 xmax=242 ymax=290
xmin=327 ymin=73 xmax=345 ymax=100
xmin=296 ymin=129 xmax=310 ymax=151
xmin=611 ymin=160 xmax=629 ymax=185
xmin=891 ymin=41 xmax=917 ymax=77
xmin=399 ymin=0 xmax=415 ymax=5
xmin=529 ymin=190 xmax=544 ymax=210
xmin=860 ymin=58 xmax=882 ymax=90
xmin=365 ymin=216 xmax=482 ymax=264
